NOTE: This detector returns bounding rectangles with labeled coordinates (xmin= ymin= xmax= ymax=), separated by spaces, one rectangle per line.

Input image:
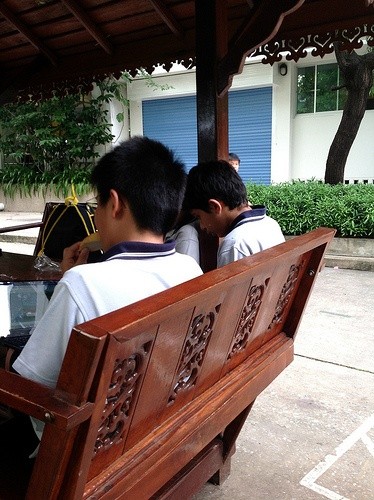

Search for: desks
xmin=0 ymin=251 xmax=64 ymax=282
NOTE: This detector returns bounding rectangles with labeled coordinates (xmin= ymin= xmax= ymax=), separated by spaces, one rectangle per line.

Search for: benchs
xmin=0 ymin=227 xmax=337 ymax=500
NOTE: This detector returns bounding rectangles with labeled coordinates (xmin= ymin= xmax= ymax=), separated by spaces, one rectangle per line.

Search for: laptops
xmin=0 ymin=280 xmax=59 ymax=352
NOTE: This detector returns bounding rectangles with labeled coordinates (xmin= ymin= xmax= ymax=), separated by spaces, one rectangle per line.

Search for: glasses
xmin=85 ymin=196 xmax=126 ymax=216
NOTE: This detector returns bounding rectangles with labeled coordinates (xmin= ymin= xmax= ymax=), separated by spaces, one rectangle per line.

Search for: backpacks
xmin=33 ymin=196 xmax=102 ymax=264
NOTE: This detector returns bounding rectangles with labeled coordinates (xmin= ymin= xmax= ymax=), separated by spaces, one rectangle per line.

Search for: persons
xmin=164 ymin=152 xmax=285 ymax=270
xmin=0 ymin=136 xmax=204 ymax=499
